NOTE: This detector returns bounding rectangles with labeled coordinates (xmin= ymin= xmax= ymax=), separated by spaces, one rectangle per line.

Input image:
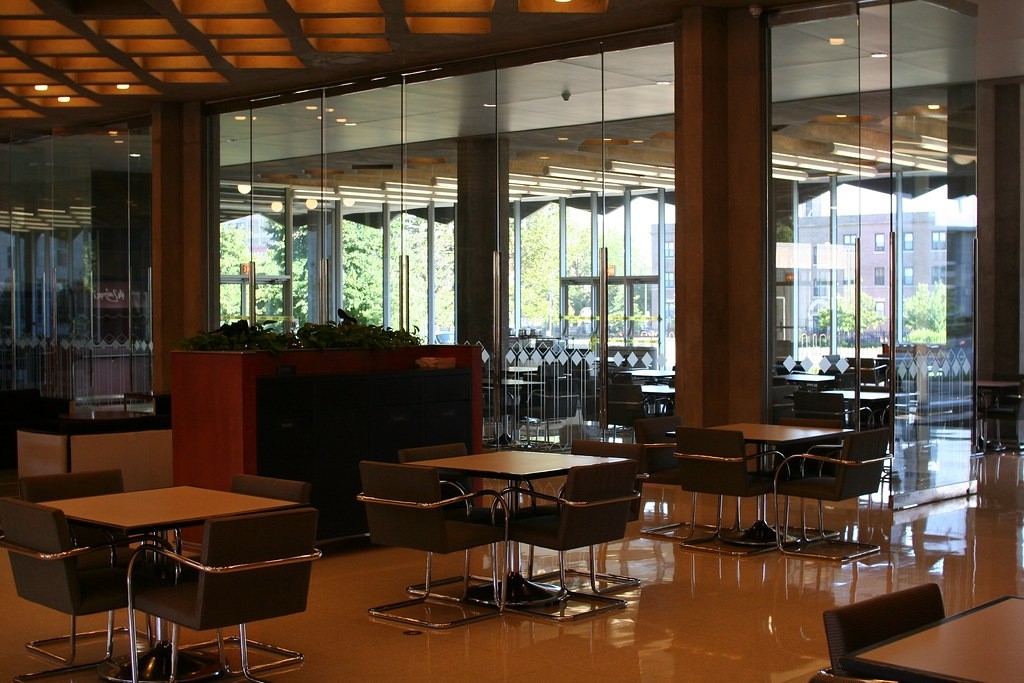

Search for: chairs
xmin=0 ymin=347 xmax=1024 ymax=683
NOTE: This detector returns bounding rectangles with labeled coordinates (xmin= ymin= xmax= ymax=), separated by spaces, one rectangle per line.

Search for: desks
xmin=703 ymin=423 xmax=855 ymax=546
xmin=483 ymin=378 xmax=546 ymax=440
xmin=945 ymin=381 xmax=1020 ymax=452
xmin=488 ymin=366 xmax=537 ymax=420
xmin=822 ymin=390 xmax=908 ymax=429
xmin=37 ymin=486 xmax=300 ymax=683
xmin=774 ymin=374 xmax=835 ymax=390
xmin=404 ymin=450 xmax=630 ymax=609
xmin=623 ymin=369 xmax=675 ymax=414
xmin=838 ymin=594 xmax=1024 ymax=683
xmin=641 ymin=385 xmax=675 ymax=415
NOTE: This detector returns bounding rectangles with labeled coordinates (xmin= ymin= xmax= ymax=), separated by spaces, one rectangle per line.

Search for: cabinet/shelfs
xmin=169 ymin=346 xmax=485 ymax=560
